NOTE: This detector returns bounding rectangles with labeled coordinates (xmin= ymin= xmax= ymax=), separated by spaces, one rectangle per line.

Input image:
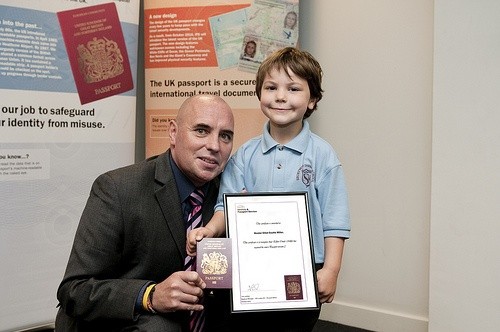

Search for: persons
xmin=244 ymin=41 xmax=257 ymax=58
xmin=185 ymin=47 xmax=349 ymax=332
xmin=284 ymin=12 xmax=297 ymax=39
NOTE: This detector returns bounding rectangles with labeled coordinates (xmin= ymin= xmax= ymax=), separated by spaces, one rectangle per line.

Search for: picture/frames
xmin=223 ymin=191 xmax=320 ymax=315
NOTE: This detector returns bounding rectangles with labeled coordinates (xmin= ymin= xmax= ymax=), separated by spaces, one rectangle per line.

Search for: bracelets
xmin=143 ymin=284 xmax=157 ymax=314
xmin=55 ymin=94 xmax=234 ymax=332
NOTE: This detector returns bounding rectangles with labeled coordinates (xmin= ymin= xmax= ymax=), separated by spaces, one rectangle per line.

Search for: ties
xmin=185 ymin=189 xmax=207 ymax=330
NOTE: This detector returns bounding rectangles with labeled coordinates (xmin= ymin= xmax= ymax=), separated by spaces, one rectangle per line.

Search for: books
xmin=196 ymin=238 xmax=232 ymax=288
